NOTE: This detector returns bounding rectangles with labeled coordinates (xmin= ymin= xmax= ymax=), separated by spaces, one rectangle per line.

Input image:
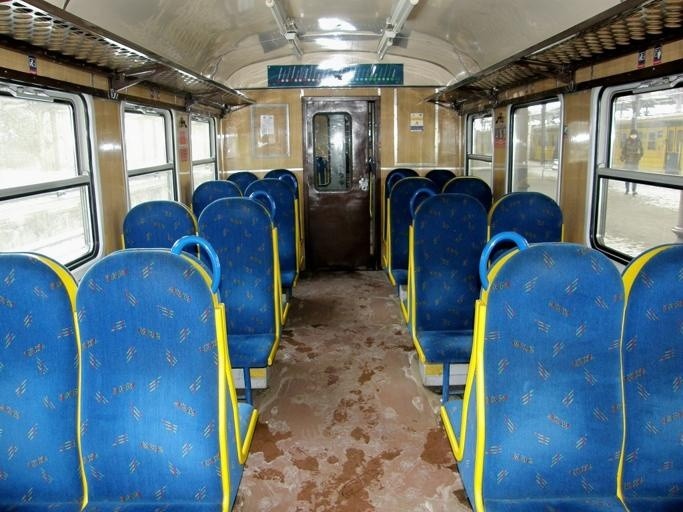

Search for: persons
xmin=619 ymin=128 xmax=643 ymax=196
